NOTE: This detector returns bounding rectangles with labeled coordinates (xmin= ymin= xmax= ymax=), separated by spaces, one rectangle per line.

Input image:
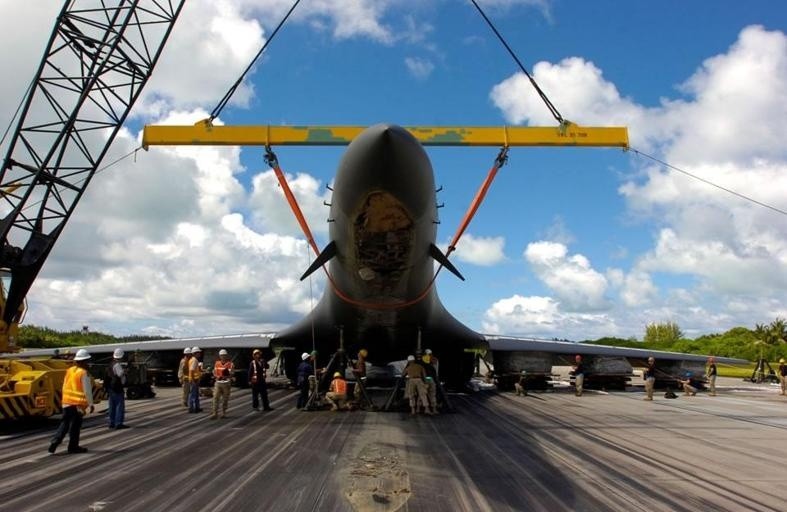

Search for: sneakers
xmin=109 ymin=423 xmax=131 ymax=430
xmin=263 ymin=407 xmax=275 ymax=411
xmin=253 ymin=407 xmax=260 ymax=412
xmin=188 ymin=407 xmax=203 ymax=414
xmin=331 ymin=405 xmax=338 ymax=411
xmin=346 ymin=402 xmax=353 ymax=410
xmin=409 ymin=410 xmax=420 ymax=417
xmin=48 ymin=442 xmax=58 ymax=453
xmin=424 ymin=409 xmax=439 ymax=417
xmin=67 ymin=446 xmax=88 ymax=454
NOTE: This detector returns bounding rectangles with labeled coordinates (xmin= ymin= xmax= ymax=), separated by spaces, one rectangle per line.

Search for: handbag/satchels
xmin=110 ymin=373 xmax=124 ymax=394
xmin=251 ymin=371 xmax=258 ymax=385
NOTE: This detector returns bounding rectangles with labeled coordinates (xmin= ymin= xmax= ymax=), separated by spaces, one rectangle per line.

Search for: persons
xmin=349 ymin=349 xmax=368 ymax=404
xmin=572 ymin=355 xmax=585 ymax=397
xmin=47 ymin=348 xmax=95 ymax=454
xmin=209 ymin=349 xmax=236 ymax=421
xmin=679 ymin=371 xmax=699 ymax=396
xmin=295 ymin=352 xmax=327 ymax=409
xmin=776 ymin=357 xmax=786 ymax=396
xmin=247 ymin=349 xmax=274 ymax=412
xmin=325 ymin=371 xmax=351 ymax=412
xmin=401 ymin=354 xmax=434 ymax=416
xmin=642 ymin=356 xmax=656 ymax=401
xmin=102 ymin=345 xmax=130 ymax=429
xmin=425 ymin=348 xmax=439 ymax=377
xmin=514 ymin=370 xmax=527 ymax=397
xmin=705 ymin=356 xmax=717 ymax=396
xmin=187 ymin=345 xmax=203 ymax=414
xmin=177 ymin=347 xmax=192 ymax=408
xmin=414 ymin=354 xmax=456 ymax=414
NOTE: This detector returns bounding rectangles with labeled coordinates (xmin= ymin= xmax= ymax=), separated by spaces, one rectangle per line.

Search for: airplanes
xmin=0 ymin=122 xmax=751 ymax=398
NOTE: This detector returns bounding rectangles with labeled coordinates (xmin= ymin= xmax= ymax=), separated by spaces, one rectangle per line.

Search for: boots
xmin=208 ymin=410 xmax=218 ymax=420
xmin=220 ymin=411 xmax=232 ymax=419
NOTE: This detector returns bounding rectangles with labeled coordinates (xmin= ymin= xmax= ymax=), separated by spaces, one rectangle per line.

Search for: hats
xmin=252 ymin=349 xmax=262 ymax=357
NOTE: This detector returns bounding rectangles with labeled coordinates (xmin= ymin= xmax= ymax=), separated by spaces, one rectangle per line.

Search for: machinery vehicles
xmin=2 ymin=1 xmax=193 ymax=421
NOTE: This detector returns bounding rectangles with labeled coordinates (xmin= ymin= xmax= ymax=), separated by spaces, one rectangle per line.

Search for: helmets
xmin=522 ymin=370 xmax=527 ymax=376
xmin=112 ymin=348 xmax=125 ymax=359
xmin=73 ymin=349 xmax=92 ymax=362
xmin=709 ymin=358 xmax=714 ymax=362
xmin=421 ymin=355 xmax=431 ymax=364
xmin=686 ymin=373 xmax=693 ymax=377
xmin=407 ymin=355 xmax=415 ymax=361
xmin=779 ymin=359 xmax=785 ymax=363
xmin=301 ymin=352 xmax=311 ymax=361
xmin=648 ymin=356 xmax=655 ymax=362
xmin=576 ymin=355 xmax=582 ymax=360
xmin=183 ymin=346 xmax=228 ymax=355
xmin=360 ymin=349 xmax=368 ymax=357
xmin=425 ymin=349 xmax=432 ymax=354
xmin=333 ymin=371 xmax=342 ymax=379
